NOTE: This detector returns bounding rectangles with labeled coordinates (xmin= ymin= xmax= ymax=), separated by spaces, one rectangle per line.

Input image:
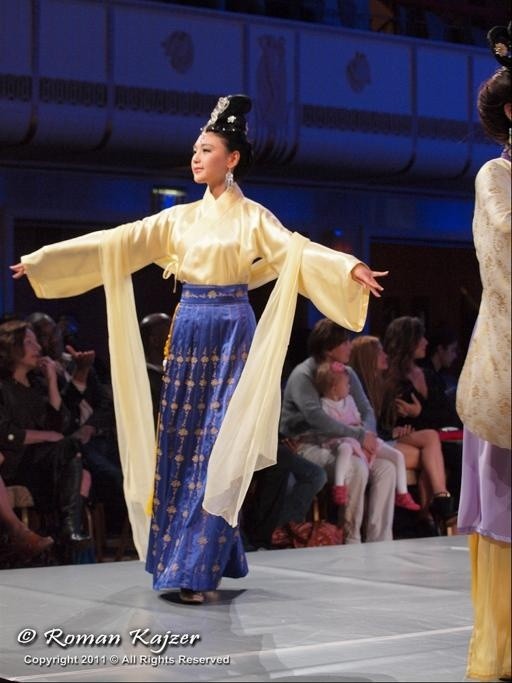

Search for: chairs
xmin=280 ymin=428 xmax=461 ymax=543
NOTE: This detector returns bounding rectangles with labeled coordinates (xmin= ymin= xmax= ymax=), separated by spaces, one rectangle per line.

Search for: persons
xmin=448 ymin=17 xmax=512 ymax=683
xmin=1 ymin=309 xmax=468 ymax=567
xmin=8 ymin=90 xmax=391 ymax=604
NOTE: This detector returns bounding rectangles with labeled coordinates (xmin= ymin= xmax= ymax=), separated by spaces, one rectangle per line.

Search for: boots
xmin=62 ymin=452 xmax=90 ymax=545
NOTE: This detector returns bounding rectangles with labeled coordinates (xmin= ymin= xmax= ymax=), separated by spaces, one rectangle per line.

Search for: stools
xmin=81 ymin=499 xmax=132 ymax=561
xmin=6 ymin=483 xmax=33 ymax=526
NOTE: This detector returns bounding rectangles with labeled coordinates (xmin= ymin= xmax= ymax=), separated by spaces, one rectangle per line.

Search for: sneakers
xmin=332 ymin=481 xmax=349 ymax=507
xmin=178 ymin=587 xmax=221 ymax=602
xmin=394 ymin=493 xmax=420 ymax=511
xmin=429 ymin=494 xmax=457 ymax=527
xmin=9 ymin=523 xmax=54 ymax=555
